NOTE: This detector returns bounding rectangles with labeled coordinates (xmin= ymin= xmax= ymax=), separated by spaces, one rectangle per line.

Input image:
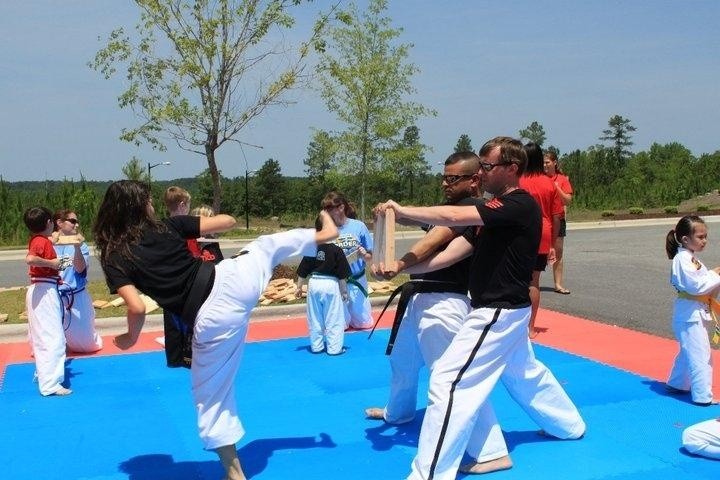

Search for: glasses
xmin=322 ymin=204 xmax=343 ymax=211
xmin=443 ymin=174 xmax=469 ymax=183
xmin=61 ymin=218 xmax=80 ymax=224
xmin=478 ymin=161 xmax=511 ymax=173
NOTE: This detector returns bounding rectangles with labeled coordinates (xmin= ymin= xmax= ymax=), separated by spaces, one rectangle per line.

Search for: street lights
xmin=147 ymin=163 xmax=169 ymax=198
xmin=245 ymin=169 xmax=261 ymax=228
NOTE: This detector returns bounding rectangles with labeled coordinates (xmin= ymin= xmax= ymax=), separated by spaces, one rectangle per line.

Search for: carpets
xmin=0 ymin=328 xmax=720 ymax=479
xmin=0 ymin=306 xmax=720 ymax=382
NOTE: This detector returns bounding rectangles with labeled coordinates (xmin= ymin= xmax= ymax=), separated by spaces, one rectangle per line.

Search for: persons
xmin=365 ymin=151 xmax=513 ymax=475
xmin=297 ymin=216 xmax=351 ymax=354
xmin=518 ymin=143 xmax=556 ymax=339
xmin=190 ymin=205 xmax=224 ymax=237
xmin=163 ymin=187 xmax=194 ymax=366
xmin=53 ymin=211 xmax=104 ymax=352
xmin=375 ymin=135 xmax=586 ymax=480
xmin=94 ymin=181 xmax=340 ymax=480
xmin=541 ymin=151 xmax=573 ymax=294
xmin=665 ymin=215 xmax=720 ymax=404
xmin=24 ymin=206 xmax=76 ymax=395
xmin=319 ymin=190 xmax=374 ymax=331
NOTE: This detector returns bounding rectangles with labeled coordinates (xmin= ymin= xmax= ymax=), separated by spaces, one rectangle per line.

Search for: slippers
xmin=554 ymin=287 xmax=571 ymax=295
